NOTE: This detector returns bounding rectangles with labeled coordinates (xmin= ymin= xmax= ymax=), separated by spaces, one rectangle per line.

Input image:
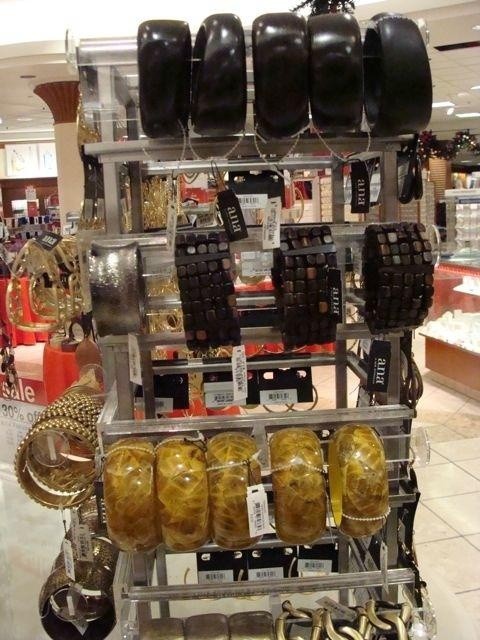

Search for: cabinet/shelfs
xmin=419 ymin=255 xmax=480 ymax=402
